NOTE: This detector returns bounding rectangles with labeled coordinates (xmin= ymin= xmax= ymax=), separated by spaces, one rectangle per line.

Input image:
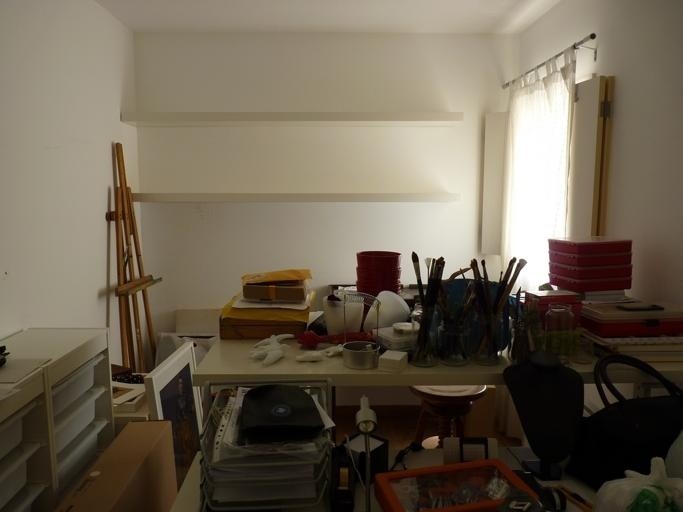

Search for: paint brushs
xmin=412 ymin=251 xmax=544 ymax=364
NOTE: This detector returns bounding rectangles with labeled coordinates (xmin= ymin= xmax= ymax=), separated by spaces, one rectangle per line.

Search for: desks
xmin=192 ymin=338 xmax=683 ymax=445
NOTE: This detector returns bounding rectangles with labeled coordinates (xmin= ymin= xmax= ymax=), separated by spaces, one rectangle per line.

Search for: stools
xmin=409 ymin=385 xmax=487 ymax=462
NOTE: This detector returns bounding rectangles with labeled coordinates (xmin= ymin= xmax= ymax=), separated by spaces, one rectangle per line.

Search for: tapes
xmin=340 ymin=467 xmax=348 ymax=489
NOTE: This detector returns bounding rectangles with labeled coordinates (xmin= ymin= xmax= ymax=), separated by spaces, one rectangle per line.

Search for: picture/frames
xmin=143 ymin=341 xmax=202 ymax=492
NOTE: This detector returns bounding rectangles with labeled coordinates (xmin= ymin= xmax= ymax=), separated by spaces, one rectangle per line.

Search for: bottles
xmin=544 ymin=302 xmax=576 ymax=358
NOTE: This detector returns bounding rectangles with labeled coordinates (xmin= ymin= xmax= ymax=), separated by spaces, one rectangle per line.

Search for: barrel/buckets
xmin=364 ymin=291 xmax=411 ymax=334
xmin=356 ymin=251 xmax=401 ymax=299
xmin=323 ymin=295 xmax=364 ymax=337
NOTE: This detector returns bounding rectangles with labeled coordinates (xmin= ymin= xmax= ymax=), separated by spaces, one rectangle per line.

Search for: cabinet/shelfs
xmin=1 ymin=326 xmax=116 ymax=512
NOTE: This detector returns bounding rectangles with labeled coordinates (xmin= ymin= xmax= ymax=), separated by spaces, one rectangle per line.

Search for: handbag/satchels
xmin=578 ymin=354 xmax=683 ymax=494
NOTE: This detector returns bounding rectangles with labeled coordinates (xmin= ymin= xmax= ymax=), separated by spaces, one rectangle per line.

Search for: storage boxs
xmin=54 ymin=420 xmax=178 ymax=512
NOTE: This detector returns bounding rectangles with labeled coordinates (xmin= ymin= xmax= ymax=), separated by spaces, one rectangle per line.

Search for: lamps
xmin=355 ymin=394 xmax=377 ymax=512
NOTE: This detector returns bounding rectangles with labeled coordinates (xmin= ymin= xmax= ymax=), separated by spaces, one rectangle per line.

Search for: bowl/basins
xmin=343 ymin=341 xmax=380 ymax=370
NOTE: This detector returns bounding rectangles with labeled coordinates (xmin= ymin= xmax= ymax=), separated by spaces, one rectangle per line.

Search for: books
xmin=219 ymin=272 xmax=311 ymax=339
xmin=209 ymin=387 xmax=337 ymax=502
xmin=602 ymin=336 xmax=683 ymax=368
